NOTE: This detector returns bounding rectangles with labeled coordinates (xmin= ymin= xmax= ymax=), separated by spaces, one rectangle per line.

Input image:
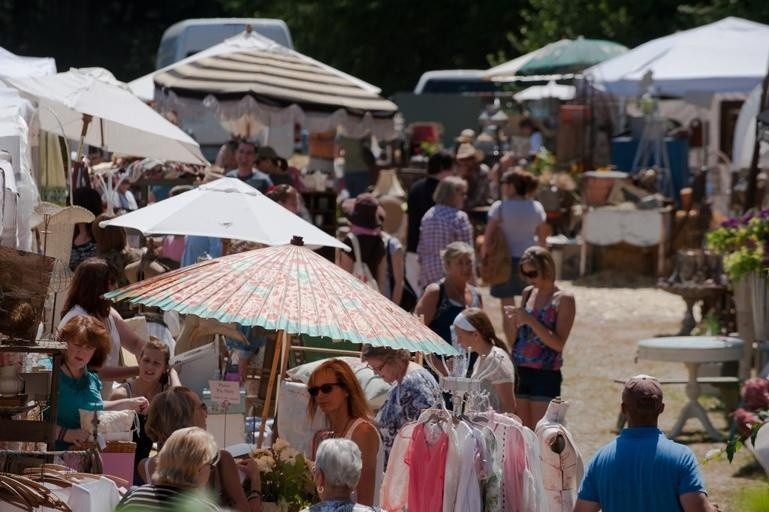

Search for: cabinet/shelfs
xmin=0 ymin=338 xmax=68 ymax=464
xmin=304 ymin=191 xmax=337 ymax=264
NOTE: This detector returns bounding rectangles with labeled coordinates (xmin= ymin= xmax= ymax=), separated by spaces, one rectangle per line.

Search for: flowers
xmin=700 ymin=374 xmax=769 ymax=467
xmin=232 ymin=437 xmax=316 ymax=500
xmin=704 ymin=206 xmax=769 ymax=280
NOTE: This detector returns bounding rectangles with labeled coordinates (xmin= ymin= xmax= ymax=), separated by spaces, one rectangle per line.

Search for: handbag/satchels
xmin=387 ymin=274 xmax=417 ymax=313
xmin=476 ymin=225 xmax=511 ymax=285
xmin=346 ymin=259 xmax=380 ymax=295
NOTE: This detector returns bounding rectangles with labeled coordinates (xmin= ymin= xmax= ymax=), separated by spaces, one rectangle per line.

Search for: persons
xmin=572 ymin=376 xmax=720 ymax=512
xmin=538 ymin=397 xmax=584 ymax=512
xmin=44 ymin=117 xmax=584 ymax=511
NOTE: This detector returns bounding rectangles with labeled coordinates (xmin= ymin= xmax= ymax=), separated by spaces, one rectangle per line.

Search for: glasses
xmin=522 ymin=268 xmax=539 ymax=279
xmin=308 ymin=382 xmax=339 ymax=396
xmin=367 ymin=358 xmax=388 ymax=371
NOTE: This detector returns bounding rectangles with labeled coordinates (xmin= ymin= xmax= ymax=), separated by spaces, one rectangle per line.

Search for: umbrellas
xmin=2 ymin=66 xmax=209 ymax=205
xmin=116 ymin=24 xmax=398 ymax=153
xmin=97 ymin=172 xmax=351 ymax=262
xmin=99 ymin=235 xmax=460 ymax=455
xmin=591 ymin=17 xmax=769 ymax=167
xmin=484 ymin=36 xmax=633 ymax=168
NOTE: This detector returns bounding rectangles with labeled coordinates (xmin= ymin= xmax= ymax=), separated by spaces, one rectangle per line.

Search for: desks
xmin=658 ymin=279 xmax=726 ymax=337
xmin=634 ymin=334 xmax=744 ymax=446
xmin=579 ymin=203 xmax=674 ymax=283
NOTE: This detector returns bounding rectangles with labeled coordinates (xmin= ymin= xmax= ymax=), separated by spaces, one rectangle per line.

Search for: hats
xmin=454 ymin=129 xmax=475 ymax=142
xmin=341 ymin=191 xmax=386 ymax=228
xmin=457 ymin=144 xmax=484 ymax=166
xmin=622 ymin=375 xmax=663 ymax=416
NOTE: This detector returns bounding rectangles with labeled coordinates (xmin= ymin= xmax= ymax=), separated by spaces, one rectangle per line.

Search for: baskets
xmin=71 ymin=441 xmax=137 ymax=453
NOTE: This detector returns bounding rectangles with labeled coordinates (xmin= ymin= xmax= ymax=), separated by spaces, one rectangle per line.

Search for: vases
xmin=731 ymin=269 xmax=769 ymax=373
xmin=259 ymin=502 xmax=281 ymax=511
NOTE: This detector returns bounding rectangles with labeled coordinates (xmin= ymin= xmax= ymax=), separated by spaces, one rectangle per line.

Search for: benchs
xmin=613 ymin=373 xmax=738 ymax=444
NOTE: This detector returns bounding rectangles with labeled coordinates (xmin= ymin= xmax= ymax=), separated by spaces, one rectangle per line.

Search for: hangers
xmin=418 ymin=390 xmax=492 ymax=418
xmin=0 ymin=463 xmax=130 ymax=511
